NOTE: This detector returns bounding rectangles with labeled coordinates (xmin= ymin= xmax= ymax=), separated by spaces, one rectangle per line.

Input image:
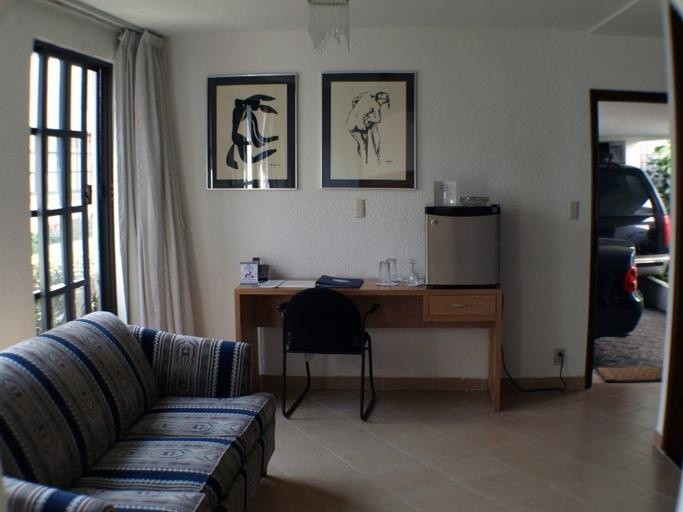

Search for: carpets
xmin=598 ymin=368 xmax=663 ymax=383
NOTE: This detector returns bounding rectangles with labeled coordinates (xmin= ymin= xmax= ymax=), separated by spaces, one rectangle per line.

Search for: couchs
xmin=0 ymin=311 xmax=275 ymax=512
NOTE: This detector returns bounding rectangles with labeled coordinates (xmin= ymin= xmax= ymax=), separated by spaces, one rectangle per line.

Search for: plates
xmin=403 ymin=282 xmax=423 ymax=286
xmin=375 ymin=282 xmax=399 ymax=287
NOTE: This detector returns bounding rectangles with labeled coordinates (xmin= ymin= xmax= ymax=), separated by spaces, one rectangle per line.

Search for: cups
xmin=386 ymin=258 xmax=397 ymax=278
xmin=378 ymin=260 xmax=390 ymax=283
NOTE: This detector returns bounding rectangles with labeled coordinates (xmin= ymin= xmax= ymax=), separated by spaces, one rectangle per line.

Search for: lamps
xmin=304 ymin=0 xmax=351 ymax=56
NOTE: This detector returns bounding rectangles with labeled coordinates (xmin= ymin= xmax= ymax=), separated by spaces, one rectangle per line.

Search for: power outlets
xmin=553 ymin=348 xmax=565 ymax=363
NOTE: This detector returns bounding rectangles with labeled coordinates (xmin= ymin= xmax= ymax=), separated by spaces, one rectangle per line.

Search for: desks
xmin=234 ymin=278 xmax=503 ymax=412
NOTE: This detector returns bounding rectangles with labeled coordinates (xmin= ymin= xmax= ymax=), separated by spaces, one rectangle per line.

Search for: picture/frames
xmin=204 ymin=72 xmax=298 ymax=191
xmin=320 ymin=70 xmax=417 ymax=191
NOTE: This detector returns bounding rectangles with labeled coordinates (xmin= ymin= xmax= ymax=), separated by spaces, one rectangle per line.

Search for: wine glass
xmin=406 ymin=259 xmax=419 ymax=284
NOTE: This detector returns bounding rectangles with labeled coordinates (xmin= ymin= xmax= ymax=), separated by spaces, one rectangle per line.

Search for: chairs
xmin=276 ymin=287 xmax=382 ymax=421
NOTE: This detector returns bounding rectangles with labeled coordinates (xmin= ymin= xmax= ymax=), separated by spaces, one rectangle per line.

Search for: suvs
xmin=596 ymin=154 xmax=670 ymax=277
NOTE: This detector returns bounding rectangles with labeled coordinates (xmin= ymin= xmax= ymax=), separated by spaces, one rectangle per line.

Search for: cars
xmin=593 ymin=239 xmax=645 ymax=340
